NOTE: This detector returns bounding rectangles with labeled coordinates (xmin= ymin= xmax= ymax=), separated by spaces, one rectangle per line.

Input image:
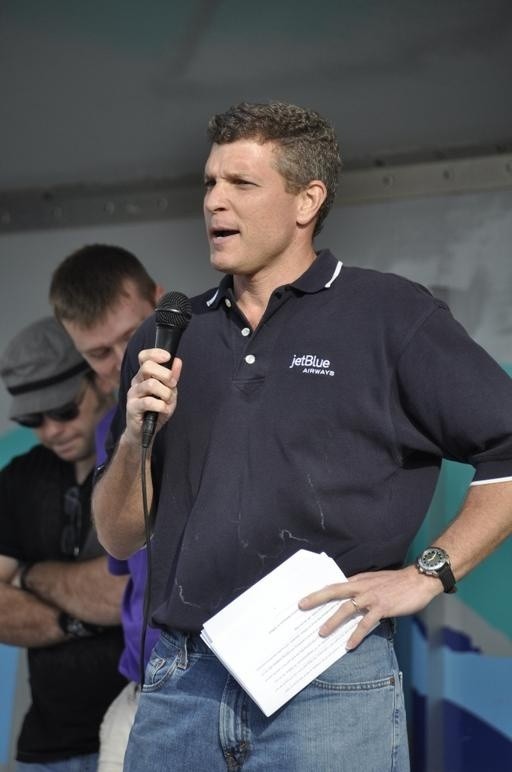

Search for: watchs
xmin=415 ymin=547 xmax=459 ymax=594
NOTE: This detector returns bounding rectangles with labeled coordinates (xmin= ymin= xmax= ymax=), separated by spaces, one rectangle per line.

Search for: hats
xmin=0 ymin=316 xmax=89 ymax=420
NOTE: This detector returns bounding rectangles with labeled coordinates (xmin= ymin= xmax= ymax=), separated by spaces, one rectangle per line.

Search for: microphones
xmin=142 ymin=290 xmax=194 ymax=448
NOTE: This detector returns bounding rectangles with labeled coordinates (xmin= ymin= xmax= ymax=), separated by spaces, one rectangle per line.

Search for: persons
xmin=92 ymin=101 xmax=512 ymax=772
xmin=1 ymin=316 xmax=131 ymax=770
xmin=51 ymin=244 xmax=175 ymax=770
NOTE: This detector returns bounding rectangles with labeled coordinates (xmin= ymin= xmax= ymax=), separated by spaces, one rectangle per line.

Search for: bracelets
xmin=56 ymin=606 xmax=94 ymax=637
xmin=10 ymin=558 xmax=35 ymax=587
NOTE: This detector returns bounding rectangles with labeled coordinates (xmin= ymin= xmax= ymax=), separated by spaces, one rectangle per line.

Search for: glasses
xmin=14 ymin=379 xmax=90 ymax=428
